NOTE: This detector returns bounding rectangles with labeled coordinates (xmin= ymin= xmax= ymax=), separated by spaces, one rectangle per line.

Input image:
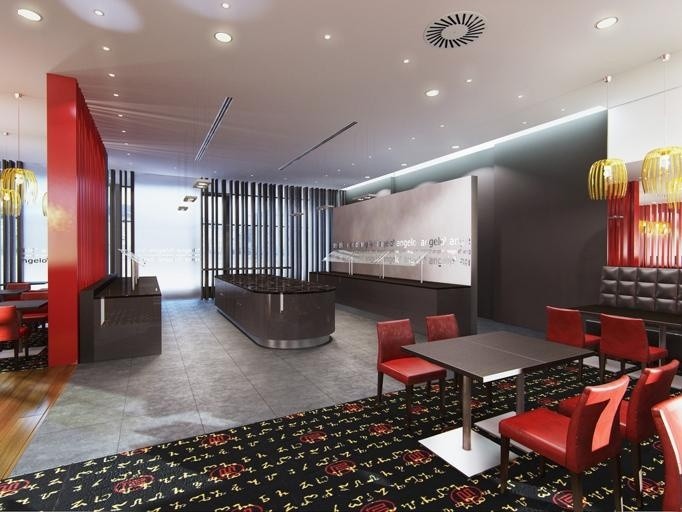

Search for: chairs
xmin=543 ymin=305 xmax=604 ymax=382
xmin=0 ymin=307 xmax=31 ymax=359
xmin=22 ymin=292 xmax=48 ymax=338
xmin=499 ymin=373 xmax=629 ymax=512
xmin=6 ymin=283 xmax=31 ymax=302
xmin=557 ymin=361 xmax=678 ymax=511
xmin=598 ymin=312 xmax=667 ymax=375
xmin=377 ymin=319 xmax=447 ymax=430
xmin=651 ymin=395 xmax=681 ymax=511
xmin=424 ymin=315 xmax=493 ymax=407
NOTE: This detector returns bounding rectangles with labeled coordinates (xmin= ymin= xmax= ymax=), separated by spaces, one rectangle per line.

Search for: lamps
xmin=193 ymin=178 xmax=210 ymax=189
xmin=641 ymin=54 xmax=682 ymax=210
xmin=0 ymin=129 xmax=21 ymax=217
xmin=587 ymin=73 xmax=628 ymax=199
xmin=0 ymin=92 xmax=38 ymax=207
xmin=183 ymin=196 xmax=197 ymax=203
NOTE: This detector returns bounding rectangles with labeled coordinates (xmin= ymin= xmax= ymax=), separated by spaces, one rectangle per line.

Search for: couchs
xmin=570 ymin=265 xmax=682 ymax=370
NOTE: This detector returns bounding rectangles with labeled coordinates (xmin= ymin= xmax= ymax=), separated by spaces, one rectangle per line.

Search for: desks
xmin=0 ymin=290 xmax=28 ymax=300
xmin=402 ymin=330 xmax=595 ymax=450
xmin=0 ymin=300 xmax=48 ymax=352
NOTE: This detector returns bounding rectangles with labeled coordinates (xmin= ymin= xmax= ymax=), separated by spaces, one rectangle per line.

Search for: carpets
xmin=0 ymin=323 xmax=48 ymax=370
xmin=0 ymin=360 xmax=682 ymax=512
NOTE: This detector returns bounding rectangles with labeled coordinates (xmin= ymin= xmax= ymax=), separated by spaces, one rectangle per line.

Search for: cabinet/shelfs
xmin=79 ymin=275 xmax=162 ymax=363
xmin=215 ymin=274 xmax=337 ymax=349
